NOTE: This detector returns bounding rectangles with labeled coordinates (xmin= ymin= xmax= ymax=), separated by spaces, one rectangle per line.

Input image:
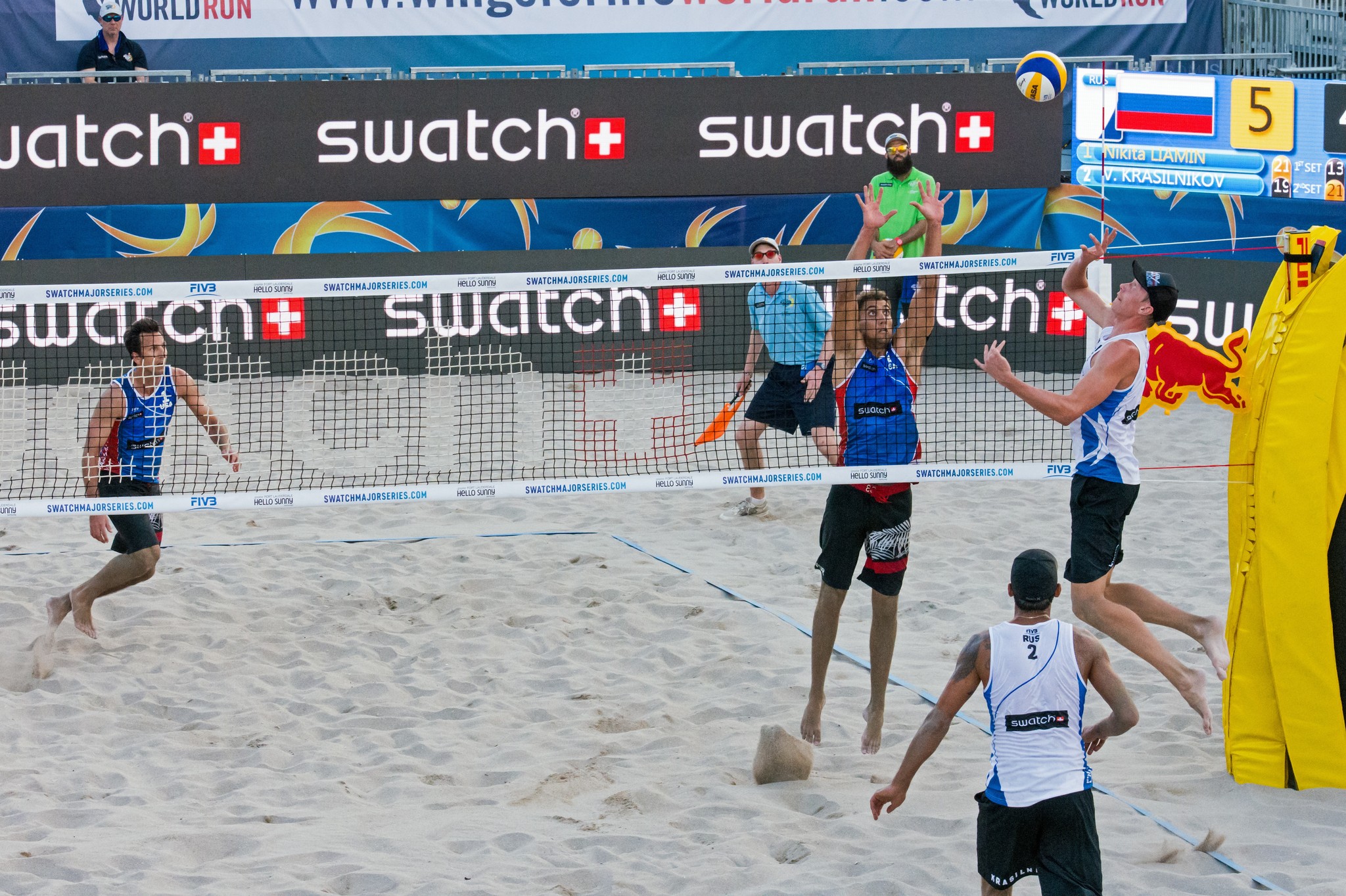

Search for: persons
xmin=77 ymin=1 xmax=150 ymax=84
xmin=973 ymin=228 xmax=1231 ymax=737
xmin=800 ymin=179 xmax=954 ymax=761
xmin=719 ymin=235 xmax=840 ymax=524
xmin=867 ymin=133 xmax=936 ymax=346
xmin=868 ymin=549 xmax=1142 ymax=896
xmin=45 ymin=318 xmax=241 ymax=641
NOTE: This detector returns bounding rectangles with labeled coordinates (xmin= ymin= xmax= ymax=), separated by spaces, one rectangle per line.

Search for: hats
xmin=100 ymin=1 xmax=122 ymax=17
xmin=749 ymin=237 xmax=780 ymax=254
xmin=1132 ymin=260 xmax=1180 ymax=322
xmin=1010 ymin=549 xmax=1058 ymax=601
xmin=884 ymin=133 xmax=910 ymax=151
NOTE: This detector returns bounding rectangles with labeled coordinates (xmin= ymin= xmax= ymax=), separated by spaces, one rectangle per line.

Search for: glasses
xmin=752 ymin=250 xmax=778 ymax=260
xmin=886 ymin=144 xmax=907 ymax=155
xmin=102 ymin=15 xmax=122 ymax=22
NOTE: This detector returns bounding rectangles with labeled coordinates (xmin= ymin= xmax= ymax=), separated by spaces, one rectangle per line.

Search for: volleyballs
xmin=1015 ymin=50 xmax=1067 ymax=102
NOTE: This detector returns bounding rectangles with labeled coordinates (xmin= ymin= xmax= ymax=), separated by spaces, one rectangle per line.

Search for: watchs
xmin=893 ymin=237 xmax=903 ymax=246
xmin=814 ymin=361 xmax=826 ymax=371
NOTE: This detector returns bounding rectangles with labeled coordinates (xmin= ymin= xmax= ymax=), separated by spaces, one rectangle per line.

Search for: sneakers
xmin=720 ymin=498 xmax=769 ymax=521
